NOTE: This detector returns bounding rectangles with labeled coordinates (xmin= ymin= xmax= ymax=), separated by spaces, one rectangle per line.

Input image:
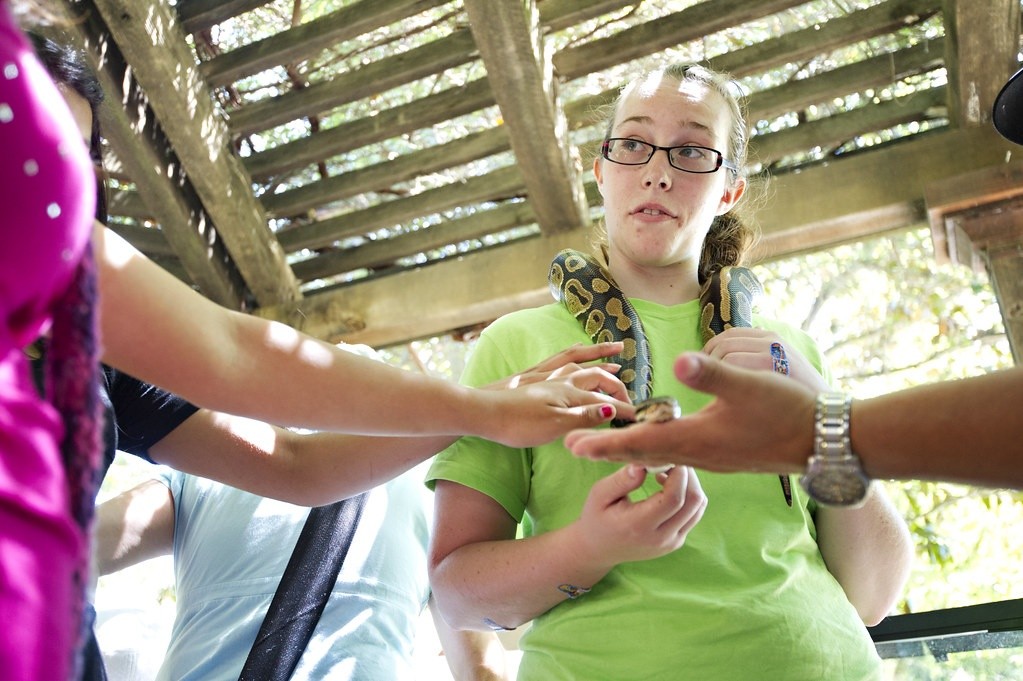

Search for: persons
xmin=424 ymin=62 xmax=915 ymax=681
xmin=565 ymin=66 xmax=1023 ymax=510
xmin=0 ymin=0 xmax=637 ymax=681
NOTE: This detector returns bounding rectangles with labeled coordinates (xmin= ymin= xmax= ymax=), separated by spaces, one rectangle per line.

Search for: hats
xmin=992 ymin=68 xmax=1023 ymax=145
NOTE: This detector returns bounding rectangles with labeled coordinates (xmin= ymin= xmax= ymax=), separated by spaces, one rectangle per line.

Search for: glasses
xmin=600 ymin=138 xmax=739 ymax=174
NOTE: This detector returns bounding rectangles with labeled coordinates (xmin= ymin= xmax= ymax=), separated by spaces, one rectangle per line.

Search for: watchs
xmin=801 ymin=392 xmax=872 ymax=510
